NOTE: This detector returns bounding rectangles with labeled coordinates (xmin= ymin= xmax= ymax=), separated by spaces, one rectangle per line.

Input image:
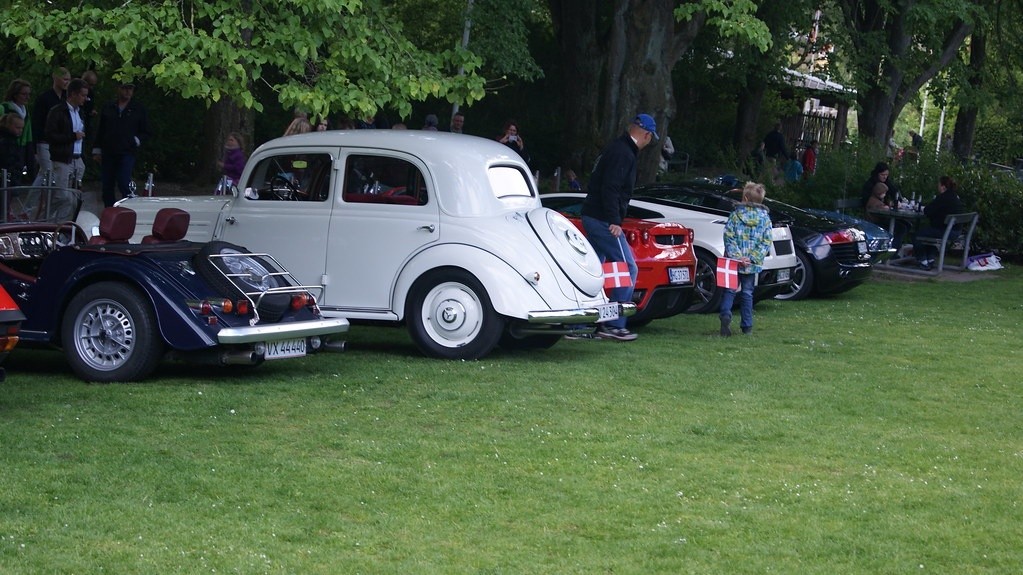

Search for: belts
xmin=74 ymin=155 xmax=81 ymax=159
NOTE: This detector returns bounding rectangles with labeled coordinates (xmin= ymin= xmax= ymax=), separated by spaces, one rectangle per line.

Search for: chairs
xmin=143 ymin=207 xmax=190 ymax=244
xmin=88 ymin=205 xmax=137 ymax=245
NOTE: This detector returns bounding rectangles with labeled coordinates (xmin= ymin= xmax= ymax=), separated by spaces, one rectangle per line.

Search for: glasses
xmin=509 ymin=129 xmax=517 ymax=132
xmin=319 ymin=123 xmax=328 ymax=127
xmin=454 ymin=119 xmax=464 ymax=122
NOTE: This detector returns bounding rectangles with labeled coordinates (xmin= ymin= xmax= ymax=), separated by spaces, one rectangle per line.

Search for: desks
xmin=866 ymin=206 xmax=924 ymax=272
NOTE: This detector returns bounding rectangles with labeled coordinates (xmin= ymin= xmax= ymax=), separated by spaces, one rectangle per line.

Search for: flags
xmin=716 ymin=258 xmax=738 ymax=289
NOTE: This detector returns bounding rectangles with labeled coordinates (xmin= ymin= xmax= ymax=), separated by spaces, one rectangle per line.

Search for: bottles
xmin=76 ymin=120 xmax=83 ymax=143
xmin=893 ymin=191 xmax=936 ymax=212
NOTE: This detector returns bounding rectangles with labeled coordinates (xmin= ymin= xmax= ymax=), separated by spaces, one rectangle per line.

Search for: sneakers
xmin=598 ymin=326 xmax=638 ymax=340
xmin=564 ymin=333 xmax=602 ymax=340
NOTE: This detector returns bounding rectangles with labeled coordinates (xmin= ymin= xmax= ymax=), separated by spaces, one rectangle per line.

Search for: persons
xmin=862 ymin=161 xmax=907 ymax=259
xmin=864 ymin=182 xmax=893 ymax=223
xmin=421 ymin=114 xmax=438 ymax=131
xmin=281 ymin=107 xmax=376 ymax=137
xmin=498 ymin=119 xmax=530 ymax=164
xmin=563 ymin=113 xmax=660 ymax=341
xmin=215 ymin=132 xmax=248 ymax=196
xmin=438 ymin=111 xmax=465 ymax=134
xmin=566 ymin=168 xmax=584 ymax=191
xmin=885 ymin=129 xmax=899 ymax=164
xmin=911 ymin=176 xmax=964 ymax=271
xmin=718 ymin=180 xmax=772 ymax=337
xmin=909 ymin=131 xmax=925 ymax=146
xmin=0 ymin=67 xmax=151 ymax=224
xmin=657 ymin=135 xmax=675 ymax=175
xmin=752 ymin=123 xmax=818 ymax=184
xmin=944 ymin=134 xmax=954 ymax=153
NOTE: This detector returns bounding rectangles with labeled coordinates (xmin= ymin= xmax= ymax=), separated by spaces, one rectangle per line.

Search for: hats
xmin=118 ymin=80 xmax=135 ymax=88
xmin=631 ymin=114 xmax=659 ymax=140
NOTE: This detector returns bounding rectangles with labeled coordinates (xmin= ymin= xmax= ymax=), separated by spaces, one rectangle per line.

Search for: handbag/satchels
xmin=213 ymin=174 xmax=240 ymax=196
xmin=662 ymin=146 xmax=672 ymax=161
xmin=967 ymin=251 xmax=1004 ymax=271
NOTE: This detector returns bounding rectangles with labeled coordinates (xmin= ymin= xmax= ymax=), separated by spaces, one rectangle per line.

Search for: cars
xmin=114 ymin=129 xmax=611 ymax=358
xmin=563 ymin=216 xmax=698 ymax=312
xmin=538 ymin=192 xmax=797 ymax=315
xmin=0 ymin=184 xmax=354 ymax=384
xmin=633 ymin=182 xmax=895 ymax=302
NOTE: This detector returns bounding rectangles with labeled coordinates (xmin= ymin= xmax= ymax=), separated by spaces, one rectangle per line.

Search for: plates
xmin=891 ymin=209 xmax=905 ymax=211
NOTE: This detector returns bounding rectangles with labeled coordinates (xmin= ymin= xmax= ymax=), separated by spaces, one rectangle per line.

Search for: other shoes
xmin=721 ymin=315 xmax=731 ymax=335
xmin=742 ymin=327 xmax=752 ymax=334
xmin=891 ymin=256 xmax=900 ymax=259
xmin=913 ymin=261 xmax=934 ymax=271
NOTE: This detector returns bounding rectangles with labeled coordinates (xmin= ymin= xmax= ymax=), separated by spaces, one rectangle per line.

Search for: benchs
xmin=917 ymin=209 xmax=978 ymax=276
xmin=837 ymin=198 xmax=870 ymax=222
